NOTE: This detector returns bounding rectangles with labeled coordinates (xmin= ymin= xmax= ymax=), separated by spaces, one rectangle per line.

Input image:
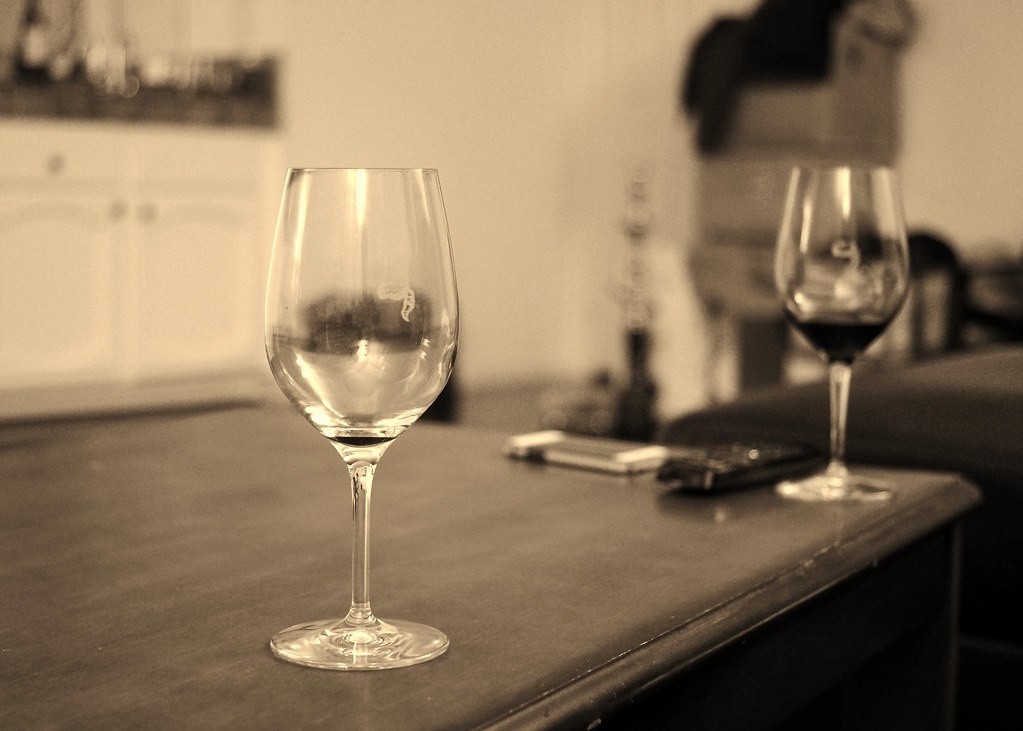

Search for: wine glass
xmin=264 ymin=167 xmax=458 ymax=670
xmin=773 ymin=165 xmax=908 ymax=503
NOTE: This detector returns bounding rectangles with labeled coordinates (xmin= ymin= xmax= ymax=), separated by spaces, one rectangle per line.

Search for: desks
xmin=0 ymin=405 xmax=977 ymax=731
xmin=656 ymin=346 xmax=1023 ymax=731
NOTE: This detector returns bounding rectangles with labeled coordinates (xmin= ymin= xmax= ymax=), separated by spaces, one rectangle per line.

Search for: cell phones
xmin=658 ymin=438 xmax=822 ymax=491
xmin=506 ymin=428 xmax=670 ymax=472
